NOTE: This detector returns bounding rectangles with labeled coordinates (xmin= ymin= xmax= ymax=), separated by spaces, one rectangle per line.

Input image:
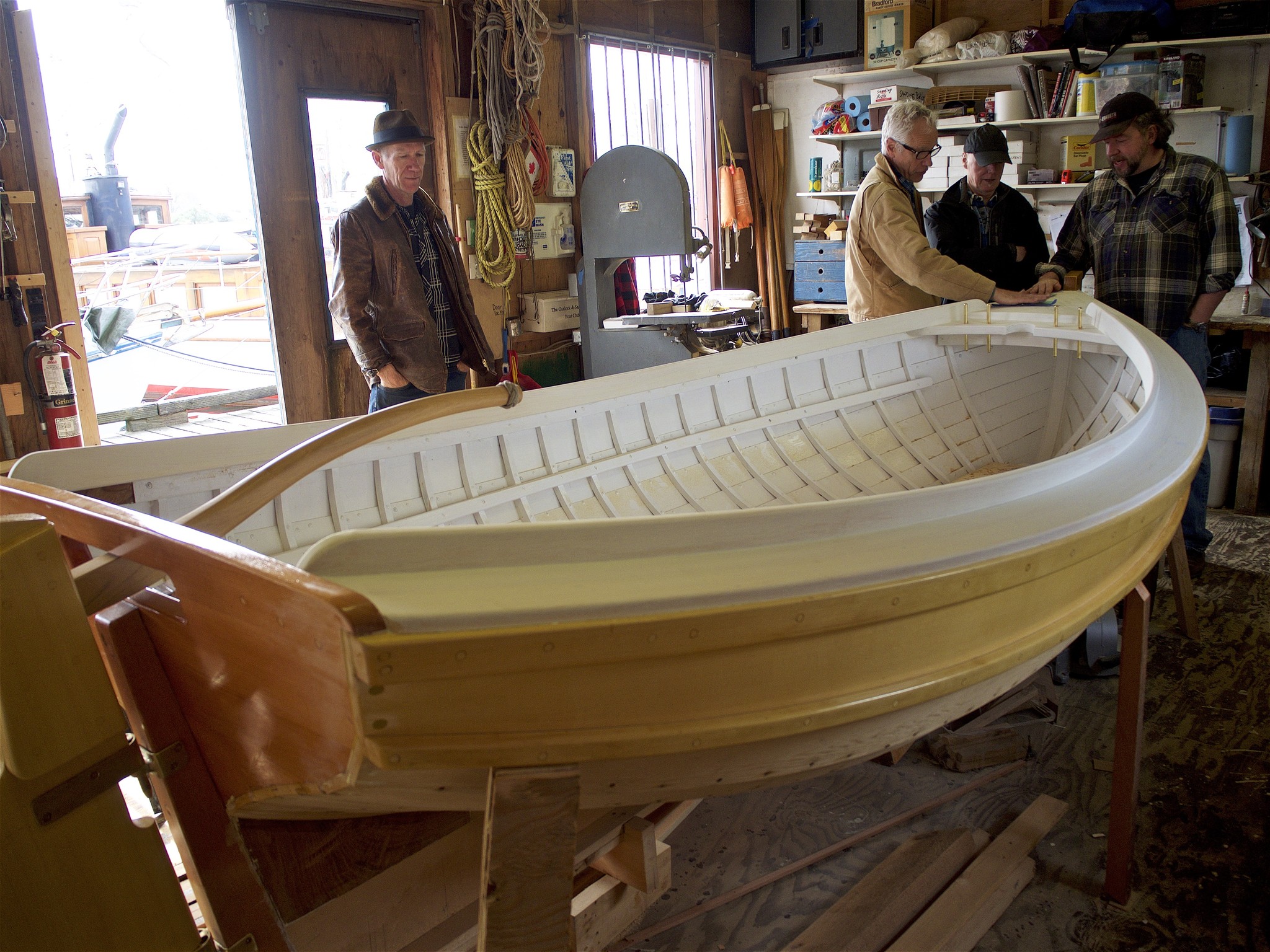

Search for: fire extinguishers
xmin=23 ymin=322 xmax=85 ymax=450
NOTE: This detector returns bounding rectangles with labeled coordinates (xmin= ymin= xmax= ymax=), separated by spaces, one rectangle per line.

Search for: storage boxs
xmin=1059 ymin=134 xmax=1096 ymax=171
xmin=1003 ymin=163 xmax=1031 ymax=175
xmin=912 ymin=129 xmax=969 ymax=189
xmin=1007 ymin=141 xmax=1038 ymax=153
xmin=1206 ymin=406 xmax=1243 ymax=508
xmin=864 ymin=0 xmax=933 ymax=71
xmin=1000 ymin=175 xmax=1028 ymax=185
xmin=1093 ymin=73 xmax=1157 ymax=114
xmin=1027 ymin=168 xmax=1056 ymax=184
xmin=1001 ymin=130 xmax=1031 ymax=141
xmin=517 ymin=290 xmax=580 ymax=333
xmin=864 ymin=101 xmax=894 ymax=131
xmin=1008 ymin=152 xmax=1036 ymax=163
xmin=870 ymin=85 xmax=929 ymax=105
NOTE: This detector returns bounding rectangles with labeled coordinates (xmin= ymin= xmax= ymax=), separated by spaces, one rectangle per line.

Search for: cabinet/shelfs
xmin=792 ymin=28 xmax=1270 ymax=241
xmin=794 ymin=239 xmax=847 ymax=303
xmin=751 ymin=0 xmax=865 ymax=74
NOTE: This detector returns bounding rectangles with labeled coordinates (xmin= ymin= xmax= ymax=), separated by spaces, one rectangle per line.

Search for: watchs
xmin=1185 ymin=318 xmax=1208 ymax=333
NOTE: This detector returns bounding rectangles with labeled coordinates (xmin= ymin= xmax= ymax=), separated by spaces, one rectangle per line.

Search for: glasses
xmin=898 ymin=142 xmax=942 ymax=160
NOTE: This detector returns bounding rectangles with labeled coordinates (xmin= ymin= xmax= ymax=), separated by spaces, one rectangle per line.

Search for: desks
xmin=1204 ymin=312 xmax=1270 ymax=517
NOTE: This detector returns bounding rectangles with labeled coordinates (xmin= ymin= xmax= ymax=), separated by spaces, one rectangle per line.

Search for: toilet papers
xmin=845 ymin=94 xmax=871 ymax=117
xmin=855 ymin=111 xmax=872 ymax=132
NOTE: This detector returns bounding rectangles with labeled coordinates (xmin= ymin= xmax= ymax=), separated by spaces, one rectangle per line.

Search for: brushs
xmin=502 ymin=327 xmax=510 ymax=376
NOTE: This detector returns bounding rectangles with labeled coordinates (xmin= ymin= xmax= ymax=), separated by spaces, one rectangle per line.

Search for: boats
xmin=4 ymin=288 xmax=1211 ymax=813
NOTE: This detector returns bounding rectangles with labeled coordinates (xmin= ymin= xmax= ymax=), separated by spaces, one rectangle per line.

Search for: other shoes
xmin=1187 ymin=552 xmax=1205 ymax=579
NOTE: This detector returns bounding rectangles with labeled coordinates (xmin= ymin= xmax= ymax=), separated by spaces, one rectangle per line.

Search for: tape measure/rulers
xmin=1061 ymin=169 xmax=1073 ymax=185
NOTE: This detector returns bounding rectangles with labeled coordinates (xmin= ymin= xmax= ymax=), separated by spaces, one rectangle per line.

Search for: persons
xmin=328 ymin=109 xmax=496 ymax=415
xmin=1025 ymin=91 xmax=1242 ymax=580
xmin=845 ymin=99 xmax=1050 ymax=324
xmin=923 ymin=123 xmax=1050 ymax=306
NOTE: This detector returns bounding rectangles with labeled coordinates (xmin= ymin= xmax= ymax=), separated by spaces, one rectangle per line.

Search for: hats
xmin=365 ymin=109 xmax=435 ymax=152
xmin=1089 ymin=92 xmax=1156 ymax=145
xmin=964 ymin=123 xmax=1012 ymax=167
